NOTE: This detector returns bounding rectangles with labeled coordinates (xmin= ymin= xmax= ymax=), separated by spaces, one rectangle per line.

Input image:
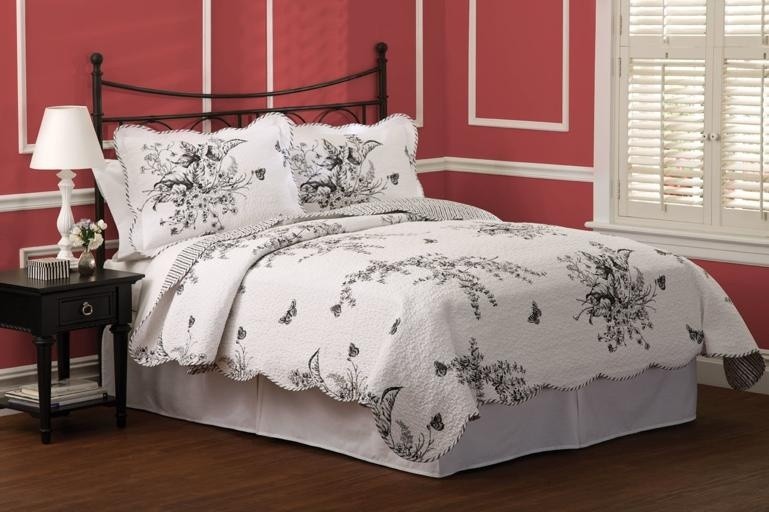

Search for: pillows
xmin=284 ymin=113 xmax=427 ymax=216
xmin=90 ymin=158 xmax=143 ymax=262
xmin=112 ymin=108 xmax=309 ymax=252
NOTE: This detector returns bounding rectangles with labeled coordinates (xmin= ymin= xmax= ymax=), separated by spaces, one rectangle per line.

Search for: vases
xmin=78 ymin=250 xmax=95 ymax=279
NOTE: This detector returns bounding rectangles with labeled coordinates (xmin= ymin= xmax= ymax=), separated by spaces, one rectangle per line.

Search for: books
xmin=5 ymin=378 xmax=108 ymax=408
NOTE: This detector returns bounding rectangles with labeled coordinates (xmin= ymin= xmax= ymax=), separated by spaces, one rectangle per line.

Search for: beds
xmin=80 ymin=40 xmax=767 ymax=481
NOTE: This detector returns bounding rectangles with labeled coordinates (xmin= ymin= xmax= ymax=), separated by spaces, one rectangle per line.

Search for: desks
xmin=0 ymin=264 xmax=146 ymax=446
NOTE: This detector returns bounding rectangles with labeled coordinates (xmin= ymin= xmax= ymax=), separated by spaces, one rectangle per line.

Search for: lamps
xmin=29 ymin=101 xmax=106 ymax=271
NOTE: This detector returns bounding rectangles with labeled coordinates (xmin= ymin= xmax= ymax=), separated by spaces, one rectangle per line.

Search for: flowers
xmin=66 ymin=217 xmax=110 ymax=253
